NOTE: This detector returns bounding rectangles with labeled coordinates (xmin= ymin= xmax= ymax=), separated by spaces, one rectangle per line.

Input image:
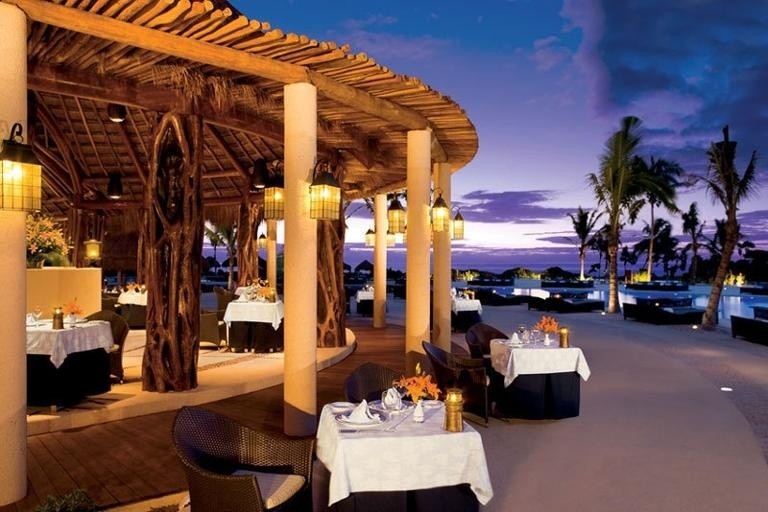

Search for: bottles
xmin=52 ymin=307 xmax=63 ymax=330
xmin=559 ymin=328 xmax=569 ymax=348
xmin=443 ymin=389 xmax=465 ymax=432
xmin=269 ymin=288 xmax=276 ymax=302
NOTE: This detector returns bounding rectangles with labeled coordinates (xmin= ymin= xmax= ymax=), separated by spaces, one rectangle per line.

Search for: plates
xmin=333 ymin=409 xmax=387 ymax=428
xmin=367 ymin=399 xmax=414 ymax=413
xmin=508 ymin=344 xmax=527 ymax=347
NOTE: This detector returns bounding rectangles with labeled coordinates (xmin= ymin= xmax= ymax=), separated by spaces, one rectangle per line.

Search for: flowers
xmin=532 ymin=315 xmax=559 ymax=334
xmin=25 ymin=214 xmax=73 ymax=265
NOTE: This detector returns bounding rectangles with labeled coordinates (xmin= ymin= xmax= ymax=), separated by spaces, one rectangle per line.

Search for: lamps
xmin=0 ymin=123 xmax=43 ymax=213
xmin=252 ymin=136 xmax=464 ymax=251
xmin=106 ymin=103 xmax=123 ymax=199
xmin=83 ymin=240 xmax=104 ymax=267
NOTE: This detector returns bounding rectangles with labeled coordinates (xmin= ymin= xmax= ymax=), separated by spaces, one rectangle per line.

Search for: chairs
xmin=25 ymin=284 xmax=128 ymax=415
xmin=199 ymin=306 xmax=226 ymax=351
xmin=213 ymin=286 xmax=241 ymax=311
xmin=344 ymin=322 xmax=509 ymax=427
xmin=171 ymin=406 xmax=316 ymax=512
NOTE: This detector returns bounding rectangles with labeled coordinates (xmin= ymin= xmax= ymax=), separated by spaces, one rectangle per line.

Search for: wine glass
xmin=530 ymin=329 xmax=540 ymax=346
xmin=32 ymin=307 xmax=43 ymax=329
xmin=381 ymin=398 xmax=400 ymax=433
xmin=246 ymin=280 xmax=265 ymax=303
xmin=518 ymin=326 xmax=526 ymax=343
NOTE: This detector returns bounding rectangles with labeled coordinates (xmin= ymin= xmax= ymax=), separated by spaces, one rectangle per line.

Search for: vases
xmin=544 ymin=333 xmax=551 ymax=346
